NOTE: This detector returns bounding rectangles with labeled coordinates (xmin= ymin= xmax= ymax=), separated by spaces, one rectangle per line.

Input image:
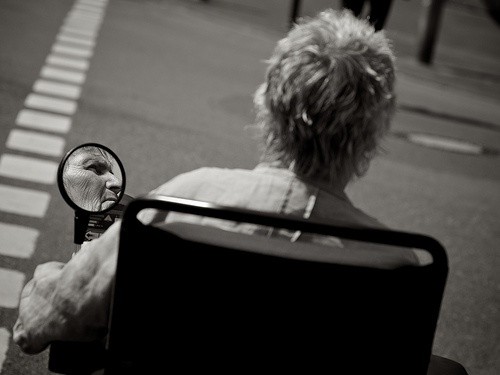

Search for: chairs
xmin=99 ymin=195 xmax=468 ymax=374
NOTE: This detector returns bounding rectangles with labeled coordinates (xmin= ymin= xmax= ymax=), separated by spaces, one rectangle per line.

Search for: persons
xmin=9 ymin=8 xmax=421 ymax=356
xmin=63 ymin=145 xmax=123 ymax=213
